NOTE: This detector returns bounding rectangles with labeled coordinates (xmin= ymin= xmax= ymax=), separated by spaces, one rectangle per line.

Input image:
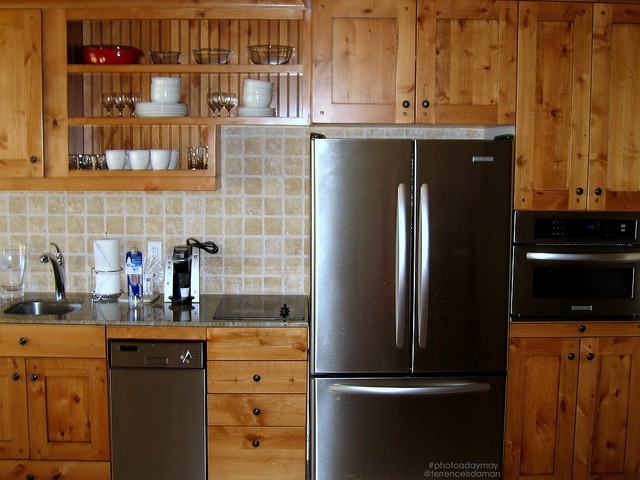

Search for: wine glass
xmin=224 ymin=89 xmax=236 ymax=117
xmin=207 ymin=88 xmax=217 ymax=117
xmin=99 ymin=88 xmax=112 ymax=117
xmin=115 ymin=88 xmax=126 ymax=120
xmin=213 ymin=93 xmax=228 ymax=116
xmin=130 ymin=88 xmax=140 ymax=115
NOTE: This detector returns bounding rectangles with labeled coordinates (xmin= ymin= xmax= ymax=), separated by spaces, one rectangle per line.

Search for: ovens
xmin=511 ymin=210 xmax=639 ymax=323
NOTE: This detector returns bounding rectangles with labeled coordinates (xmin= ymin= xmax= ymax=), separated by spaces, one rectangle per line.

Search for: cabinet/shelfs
xmin=515 ymin=0 xmax=640 ymax=212
xmin=0 ymin=324 xmax=111 ymax=476
xmin=206 ymin=328 xmax=306 ymax=476
xmin=0 ymin=6 xmax=45 ymax=187
xmin=509 ymin=322 xmax=639 ymax=474
xmin=310 ymin=4 xmax=515 ymax=125
xmin=52 ymin=2 xmax=313 ymax=193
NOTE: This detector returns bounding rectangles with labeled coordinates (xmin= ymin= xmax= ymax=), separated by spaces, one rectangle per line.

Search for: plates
xmin=239 ymin=105 xmax=279 ymax=117
xmin=136 ymin=102 xmax=189 ymax=118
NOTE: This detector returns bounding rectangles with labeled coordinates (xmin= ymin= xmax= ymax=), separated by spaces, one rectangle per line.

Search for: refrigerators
xmin=310 ymin=123 xmax=514 ymax=476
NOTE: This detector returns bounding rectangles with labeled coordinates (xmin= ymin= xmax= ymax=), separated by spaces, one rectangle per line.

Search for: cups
xmin=149 ymin=149 xmax=173 ymax=168
xmin=103 ymin=148 xmax=126 ymax=169
xmin=186 ymin=145 xmax=207 ymax=170
xmin=127 ymin=148 xmax=150 ymax=168
xmin=0 ymin=242 xmax=25 ymax=298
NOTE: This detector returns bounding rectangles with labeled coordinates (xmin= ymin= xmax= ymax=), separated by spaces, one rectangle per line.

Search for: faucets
xmin=39 ymin=241 xmax=65 ymax=301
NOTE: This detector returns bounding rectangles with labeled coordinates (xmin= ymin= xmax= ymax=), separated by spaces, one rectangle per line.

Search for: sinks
xmin=3 ymin=299 xmax=85 ymax=316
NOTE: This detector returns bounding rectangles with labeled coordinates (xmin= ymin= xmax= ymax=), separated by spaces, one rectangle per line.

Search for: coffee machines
xmin=162 ymin=245 xmax=200 ymax=309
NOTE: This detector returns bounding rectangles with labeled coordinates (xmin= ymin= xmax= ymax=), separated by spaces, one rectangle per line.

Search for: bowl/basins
xmin=148 ymin=76 xmax=181 ymax=102
xmin=194 ymin=48 xmax=231 ymax=62
xmin=240 ymin=77 xmax=277 ymax=101
xmin=83 ymin=43 xmax=141 ymax=64
xmin=249 ymin=45 xmax=291 ymax=65
xmin=149 ymin=51 xmax=182 ymax=65
